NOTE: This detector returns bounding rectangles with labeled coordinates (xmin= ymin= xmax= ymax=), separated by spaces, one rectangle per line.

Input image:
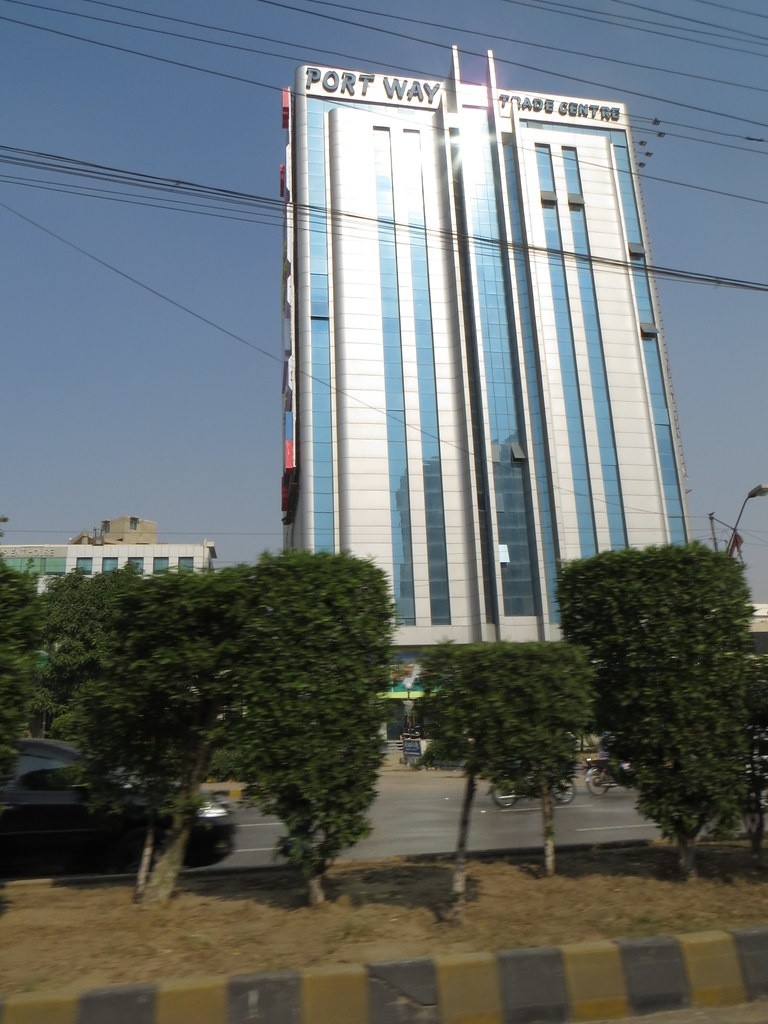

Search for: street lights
xmin=707 ymin=484 xmax=768 ymax=557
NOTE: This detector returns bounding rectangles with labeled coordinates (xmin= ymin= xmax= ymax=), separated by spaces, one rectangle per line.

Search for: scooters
xmin=582 ymin=754 xmax=639 ymax=798
xmin=484 ymin=768 xmax=577 ymax=809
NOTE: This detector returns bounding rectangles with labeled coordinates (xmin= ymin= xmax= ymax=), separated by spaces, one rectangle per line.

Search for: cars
xmin=0 ymin=738 xmax=237 ymax=874
